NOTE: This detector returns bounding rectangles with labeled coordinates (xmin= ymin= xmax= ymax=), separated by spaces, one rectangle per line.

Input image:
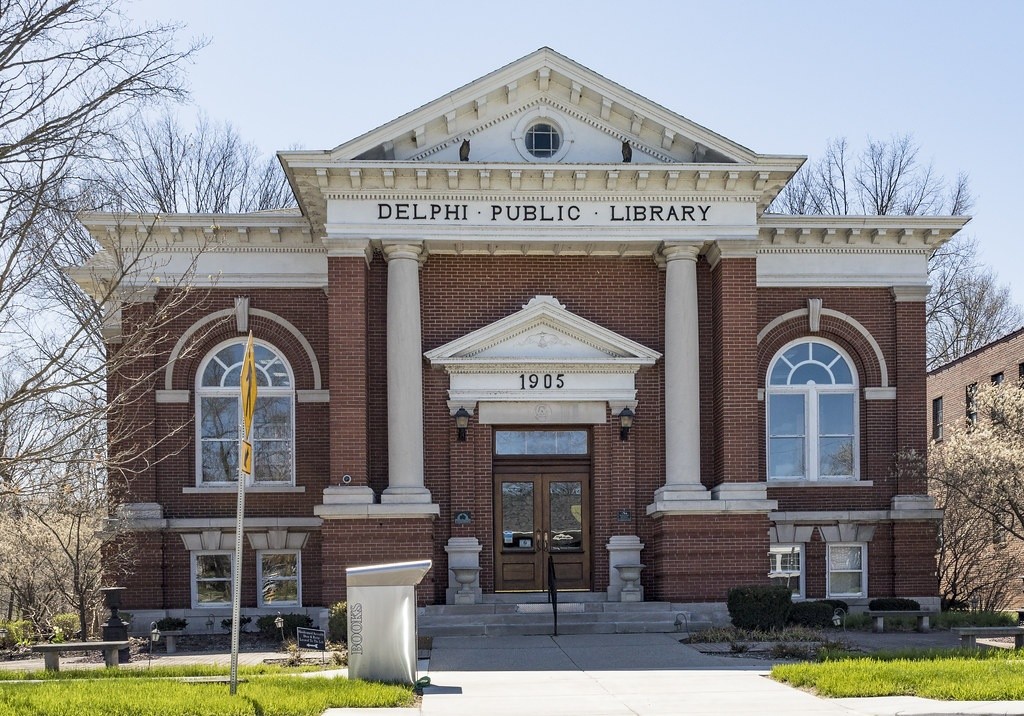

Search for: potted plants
xmin=152 ymin=617 xmax=189 ymax=653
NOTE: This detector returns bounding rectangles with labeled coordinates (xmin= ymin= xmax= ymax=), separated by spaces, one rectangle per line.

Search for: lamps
xmin=205 ymin=614 xmax=216 ymax=636
xmin=970 ymin=596 xmax=979 ymax=612
xmin=674 ymin=612 xmax=692 ymax=643
xmin=454 ymin=406 xmax=470 ymax=442
xmin=618 ymin=406 xmax=635 ymax=440
xmin=150 ymin=621 xmax=161 ymax=655
xmin=832 ymin=608 xmax=846 ymax=634
xmin=274 ymin=611 xmax=286 ymax=641
xmin=951 ymin=627 xmax=1024 ymax=649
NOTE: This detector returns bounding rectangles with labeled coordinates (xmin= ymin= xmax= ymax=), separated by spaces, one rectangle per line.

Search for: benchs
xmin=31 ymin=640 xmax=129 ymax=672
xmin=863 ymin=611 xmax=937 ymax=634
